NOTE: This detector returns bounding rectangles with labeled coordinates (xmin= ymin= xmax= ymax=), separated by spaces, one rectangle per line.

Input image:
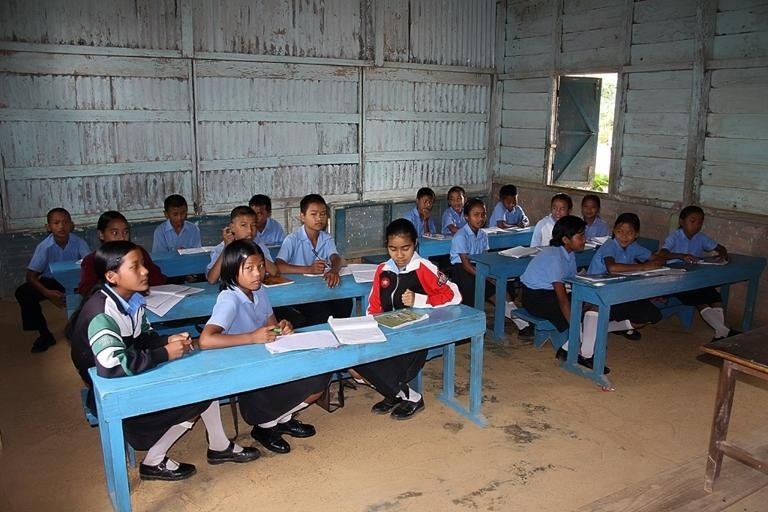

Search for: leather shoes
xmin=613 ymin=329 xmax=642 ymax=340
xmin=390 ymin=393 xmax=425 ymax=420
xmin=207 ymin=442 xmax=261 ymax=465
xmin=555 ymin=346 xmax=567 ymax=362
xmin=278 ymin=420 xmax=315 ymax=437
xmin=371 ymin=397 xmax=402 ymax=414
xmin=518 ymin=323 xmax=534 ymax=339
xmin=139 ymin=456 xmax=195 ymax=481
xmin=577 ymin=353 xmax=610 ymax=374
xmin=250 ymin=425 xmax=290 ymax=454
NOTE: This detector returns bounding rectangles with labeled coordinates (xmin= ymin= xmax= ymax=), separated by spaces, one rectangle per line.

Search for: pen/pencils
xmin=518 ymin=218 xmax=527 ymax=227
xmin=312 ymin=249 xmax=332 ymax=269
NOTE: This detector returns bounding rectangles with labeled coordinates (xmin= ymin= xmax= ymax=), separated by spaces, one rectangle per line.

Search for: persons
xmin=403 ymin=187 xmax=436 ymax=236
xmin=530 ymin=191 xmax=571 ymax=247
xmin=74 ymin=210 xmax=168 ymax=297
xmin=489 ymin=183 xmax=531 ymax=228
xmin=16 ymin=207 xmax=95 ymax=352
xmin=658 ymin=205 xmax=742 ymax=342
xmin=198 ymin=238 xmax=332 ymax=454
xmin=153 ymin=194 xmax=204 ymax=282
xmin=274 ymin=195 xmax=353 ymax=324
xmin=449 ymin=197 xmax=535 ymax=341
xmin=249 ymin=195 xmax=285 ymax=243
xmin=586 ymin=212 xmax=668 ymax=341
xmin=347 ymin=218 xmax=465 ymax=420
xmin=442 ymin=185 xmax=468 ymax=236
xmin=206 ymin=206 xmax=278 ymax=283
xmin=577 ymin=195 xmax=608 ymax=238
xmin=519 ymin=215 xmax=611 ymax=374
xmin=62 ymin=241 xmax=262 ymax=481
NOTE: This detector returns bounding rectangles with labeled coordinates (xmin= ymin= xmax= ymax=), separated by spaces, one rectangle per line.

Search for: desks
xmin=84 ymin=304 xmax=489 ymax=511
xmin=470 ymin=238 xmax=660 ymax=346
xmin=560 ymin=252 xmax=765 ymax=390
xmin=48 ymin=243 xmax=285 ymax=321
xmin=694 ymin=324 xmax=767 ymax=494
xmin=414 ymin=223 xmax=535 ymax=270
xmin=63 ymin=259 xmax=373 ymax=327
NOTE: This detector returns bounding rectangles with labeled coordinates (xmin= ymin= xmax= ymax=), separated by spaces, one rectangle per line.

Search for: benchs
xmin=80 ymin=345 xmax=444 ymax=467
xmin=510 ymin=288 xmax=695 ymax=352
xmin=153 ymin=319 xmax=209 ymax=340
xmin=361 ymin=253 xmax=392 ymax=264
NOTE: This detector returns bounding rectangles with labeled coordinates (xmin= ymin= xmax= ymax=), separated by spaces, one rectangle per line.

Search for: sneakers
xmin=31 ymin=332 xmax=56 ymax=355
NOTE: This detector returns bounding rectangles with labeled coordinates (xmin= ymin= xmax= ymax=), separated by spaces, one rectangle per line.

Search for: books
xmin=261 ymin=275 xmax=293 ymax=288
xmin=326 ymin=315 xmax=388 ymax=345
xmin=304 ymin=265 xmax=351 ymax=278
xmin=139 ymin=281 xmax=190 ymax=309
xmin=349 ymin=261 xmax=377 ymax=283
xmin=176 ymin=245 xmax=220 ymax=254
xmin=584 ymin=236 xmax=610 ymax=247
xmin=143 ymin=286 xmax=207 ymax=318
xmin=373 ymin=309 xmax=430 ymax=327
xmin=575 ymin=273 xmax=626 ymax=282
xmin=497 ymin=245 xmax=537 ymax=259
xmin=263 ymin=328 xmax=340 ymax=356
